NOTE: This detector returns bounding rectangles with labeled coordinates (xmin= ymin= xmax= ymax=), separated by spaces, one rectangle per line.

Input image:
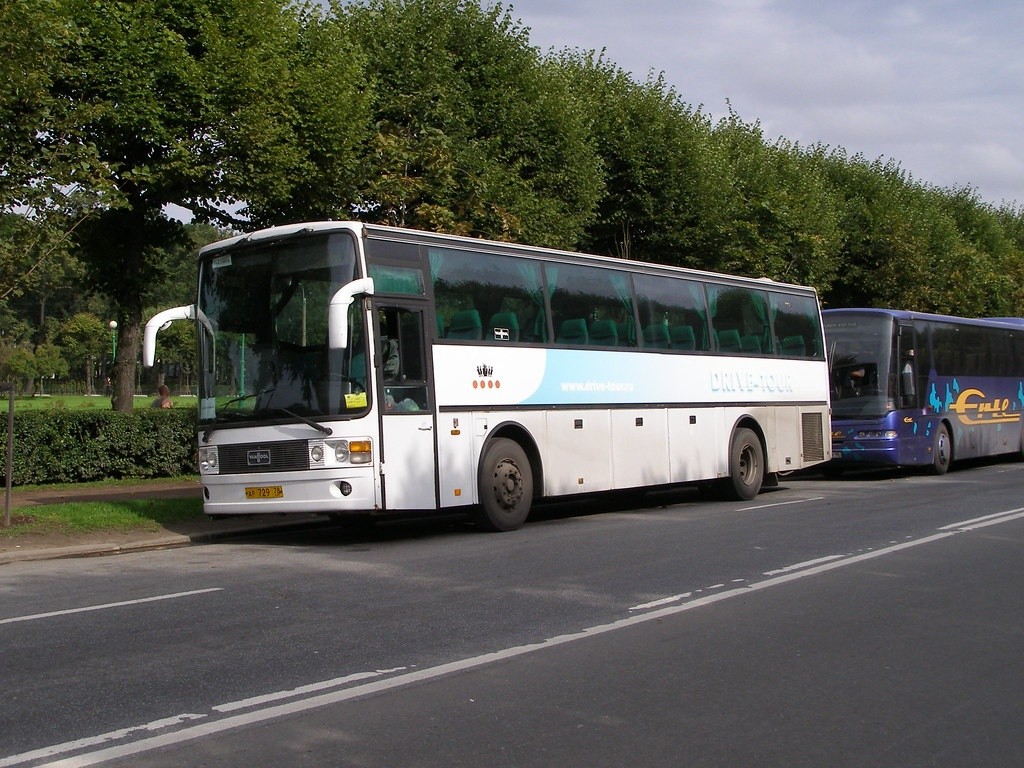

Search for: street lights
xmin=110 ymin=320 xmax=119 ymax=363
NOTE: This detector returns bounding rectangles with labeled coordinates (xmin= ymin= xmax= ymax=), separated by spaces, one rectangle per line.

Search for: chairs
xmin=435 ymin=307 xmax=807 ymax=356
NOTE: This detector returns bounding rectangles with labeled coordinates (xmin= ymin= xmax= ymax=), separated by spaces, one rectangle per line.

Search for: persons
xmin=152 ymin=384 xmax=172 ymax=408
xmin=348 ymin=320 xmax=399 ymax=390
xmin=104 ymin=378 xmax=111 ymax=397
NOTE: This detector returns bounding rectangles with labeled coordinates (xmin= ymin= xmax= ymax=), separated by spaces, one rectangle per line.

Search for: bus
xmin=775 ymin=308 xmax=1024 ymax=476
xmin=141 ymin=220 xmax=833 ymax=534
